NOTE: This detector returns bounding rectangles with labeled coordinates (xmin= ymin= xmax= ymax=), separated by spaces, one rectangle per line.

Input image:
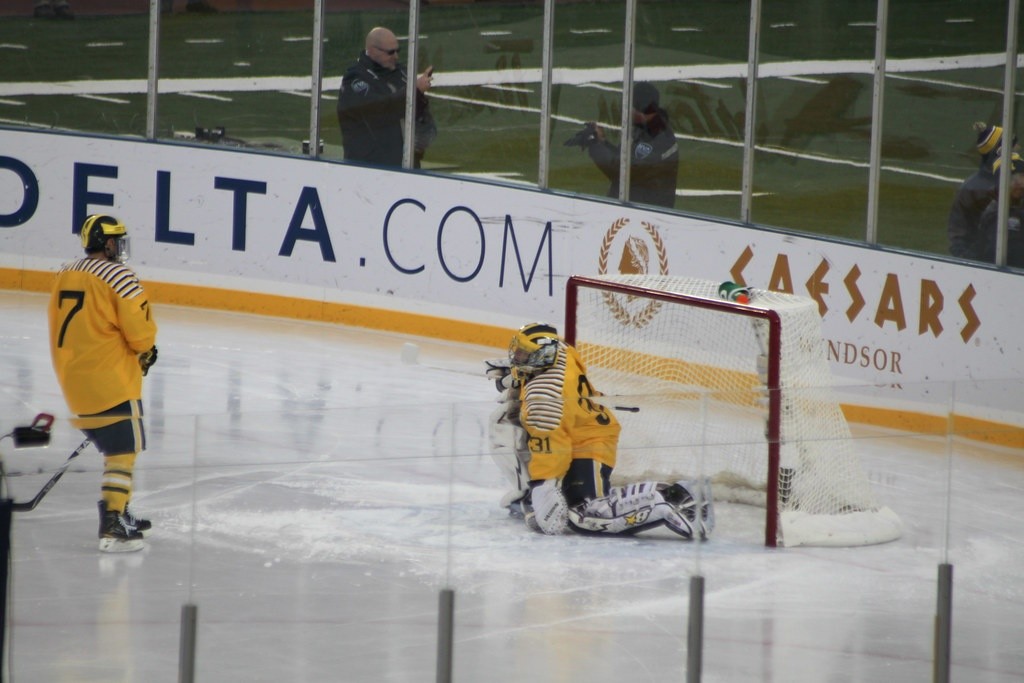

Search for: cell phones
xmin=429 ymin=68 xmax=433 ymax=78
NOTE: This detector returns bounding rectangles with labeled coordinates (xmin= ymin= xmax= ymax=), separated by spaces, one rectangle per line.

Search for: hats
xmin=992 ymin=146 xmax=1024 ymax=184
xmin=973 ymin=122 xmax=1017 ymax=160
xmin=632 ymin=81 xmax=659 ymax=113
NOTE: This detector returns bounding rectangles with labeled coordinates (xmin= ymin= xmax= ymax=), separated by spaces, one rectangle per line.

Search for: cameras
xmin=565 ymin=122 xmax=598 ymax=148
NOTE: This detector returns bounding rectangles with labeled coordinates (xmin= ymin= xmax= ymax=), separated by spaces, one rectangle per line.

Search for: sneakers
xmin=97 ymin=500 xmax=144 ymax=552
xmin=122 ymin=502 xmax=151 ymax=531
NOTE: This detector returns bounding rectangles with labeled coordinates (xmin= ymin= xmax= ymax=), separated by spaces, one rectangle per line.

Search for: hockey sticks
xmin=10 ymin=349 xmax=154 ymax=512
xmin=606 ymin=403 xmax=640 ymax=413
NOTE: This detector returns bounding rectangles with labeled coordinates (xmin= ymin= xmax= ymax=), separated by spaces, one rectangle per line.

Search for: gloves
xmin=139 ymin=345 xmax=158 ymax=370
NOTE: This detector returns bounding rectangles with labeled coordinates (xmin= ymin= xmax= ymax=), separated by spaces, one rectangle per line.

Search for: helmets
xmin=80 ymin=214 xmax=128 ymax=249
xmin=509 ymin=321 xmax=558 ymax=374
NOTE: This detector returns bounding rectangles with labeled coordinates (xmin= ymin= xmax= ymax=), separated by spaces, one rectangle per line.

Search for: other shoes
xmin=667 ymin=481 xmax=708 ymax=539
xmin=501 ymin=488 xmax=531 ymax=518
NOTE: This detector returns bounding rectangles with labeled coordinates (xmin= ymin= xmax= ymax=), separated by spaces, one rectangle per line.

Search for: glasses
xmin=374 ymin=46 xmax=401 ymax=55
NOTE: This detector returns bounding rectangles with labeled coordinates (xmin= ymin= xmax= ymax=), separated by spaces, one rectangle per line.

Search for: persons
xmin=337 ymin=27 xmax=437 ymax=171
xmin=487 ymin=323 xmax=717 ymax=541
xmin=49 ymin=214 xmax=158 ymax=553
xmin=948 ymin=119 xmax=1024 ymax=270
xmin=567 ymin=82 xmax=679 ymax=210
